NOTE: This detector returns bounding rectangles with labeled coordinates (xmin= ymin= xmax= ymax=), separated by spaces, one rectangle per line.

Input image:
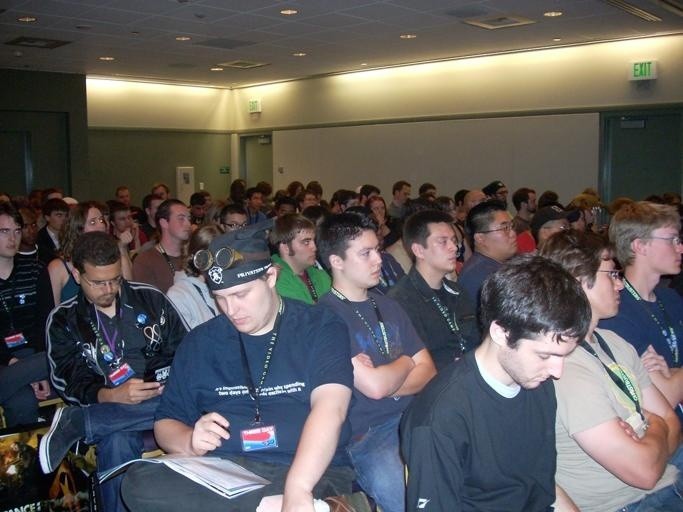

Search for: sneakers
xmin=39 ymin=403 xmax=84 ymax=474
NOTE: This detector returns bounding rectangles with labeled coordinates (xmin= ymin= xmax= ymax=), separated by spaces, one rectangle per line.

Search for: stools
xmin=0 ymin=389 xmax=67 ymax=428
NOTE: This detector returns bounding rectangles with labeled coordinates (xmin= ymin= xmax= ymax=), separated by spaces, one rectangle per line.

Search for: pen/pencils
xmin=201 ymin=410 xmax=230 ymax=434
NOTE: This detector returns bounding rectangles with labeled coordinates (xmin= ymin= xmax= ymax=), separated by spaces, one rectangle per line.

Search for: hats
xmin=528 ymin=203 xmax=584 ymax=234
xmin=191 ymin=218 xmax=274 ymax=291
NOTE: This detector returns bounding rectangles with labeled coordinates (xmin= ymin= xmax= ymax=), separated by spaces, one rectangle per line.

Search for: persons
xmin=0 ymin=179 xmax=682 ymax=512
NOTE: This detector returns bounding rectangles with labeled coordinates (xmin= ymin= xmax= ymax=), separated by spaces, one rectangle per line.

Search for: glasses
xmin=596 ymin=265 xmax=622 ymax=280
xmin=192 ymin=245 xmax=242 ymax=273
xmin=644 ymin=232 xmax=681 ymax=243
xmin=486 ymin=223 xmax=517 ymax=233
